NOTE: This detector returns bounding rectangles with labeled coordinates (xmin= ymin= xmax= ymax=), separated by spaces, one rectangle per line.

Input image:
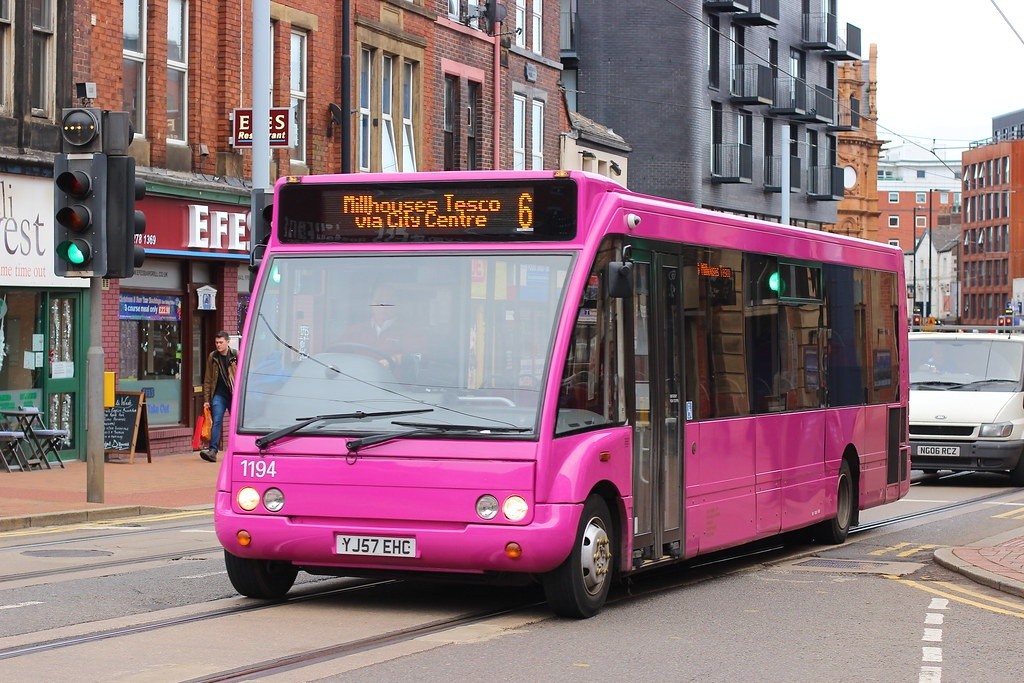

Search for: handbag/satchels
xmin=201 ymin=405 xmax=213 ymax=442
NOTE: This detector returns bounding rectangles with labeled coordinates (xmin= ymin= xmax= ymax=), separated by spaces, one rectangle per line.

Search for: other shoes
xmin=200 ymin=447 xmax=218 ymax=461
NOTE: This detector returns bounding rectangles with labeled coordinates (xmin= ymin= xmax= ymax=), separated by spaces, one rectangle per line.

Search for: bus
xmin=214 ymin=171 xmax=914 ymax=623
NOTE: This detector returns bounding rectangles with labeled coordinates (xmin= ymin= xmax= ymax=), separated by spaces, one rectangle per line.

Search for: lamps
xmin=578 ymin=150 xmax=597 ymax=160
xmin=609 ymin=160 xmax=621 ymax=176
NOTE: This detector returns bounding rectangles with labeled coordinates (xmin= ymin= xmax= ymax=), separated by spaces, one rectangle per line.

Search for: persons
xmin=920 ymin=347 xmax=946 ymax=371
xmin=335 ymin=284 xmax=427 ymax=381
xmin=200 ymin=331 xmax=240 ymax=463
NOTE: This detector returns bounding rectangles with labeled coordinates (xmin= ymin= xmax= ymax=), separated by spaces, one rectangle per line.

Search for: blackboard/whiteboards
xmin=104 ymin=391 xmax=151 ymax=454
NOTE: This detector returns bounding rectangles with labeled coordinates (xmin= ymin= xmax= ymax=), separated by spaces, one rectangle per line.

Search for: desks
xmin=0 ymin=411 xmax=65 ymax=469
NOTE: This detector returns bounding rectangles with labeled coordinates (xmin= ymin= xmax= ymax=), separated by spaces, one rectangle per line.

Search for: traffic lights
xmin=997 ymin=316 xmax=1005 ymax=333
xmin=100 ymin=111 xmax=134 ymax=157
xmin=61 ymin=106 xmax=102 ymax=153
xmin=911 ymin=315 xmax=921 ymax=332
xmin=107 ymin=156 xmax=146 ymax=278
xmin=52 ymin=153 xmax=107 ymax=278
xmin=1005 ymin=316 xmax=1013 ymax=333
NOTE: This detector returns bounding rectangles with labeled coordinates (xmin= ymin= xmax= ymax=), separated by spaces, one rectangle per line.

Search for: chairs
xmin=19 ymin=405 xmax=68 ymax=471
xmin=0 ymin=423 xmax=25 ymax=473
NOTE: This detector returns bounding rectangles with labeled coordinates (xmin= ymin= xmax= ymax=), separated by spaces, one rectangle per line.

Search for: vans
xmin=906 ymin=335 xmax=1024 ymax=489
